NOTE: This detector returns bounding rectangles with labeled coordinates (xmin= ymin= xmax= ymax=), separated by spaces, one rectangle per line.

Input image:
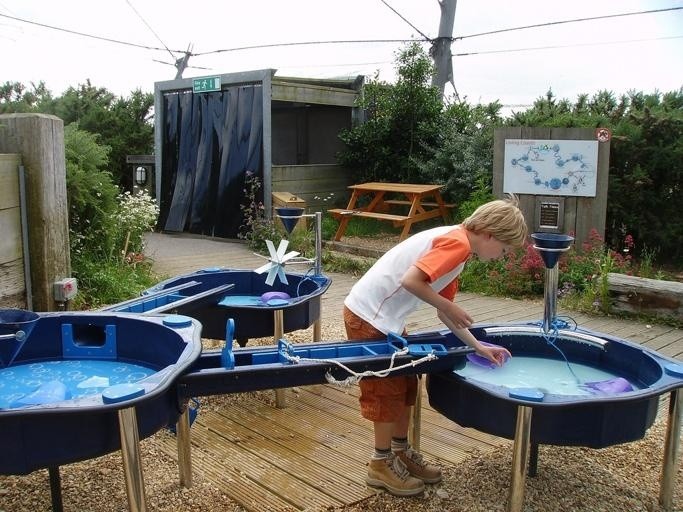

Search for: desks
xmin=334 ymin=182 xmax=450 ymax=244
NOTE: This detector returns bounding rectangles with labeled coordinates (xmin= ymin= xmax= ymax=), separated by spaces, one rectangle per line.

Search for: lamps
xmin=136 ymin=167 xmax=147 ymax=185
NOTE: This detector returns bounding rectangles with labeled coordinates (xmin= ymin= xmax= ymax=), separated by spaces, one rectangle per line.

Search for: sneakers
xmin=365 ymin=444 xmax=442 ymax=496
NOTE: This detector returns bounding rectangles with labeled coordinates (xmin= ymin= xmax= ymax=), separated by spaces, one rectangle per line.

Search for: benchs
xmin=327 ymin=200 xmax=459 ymax=245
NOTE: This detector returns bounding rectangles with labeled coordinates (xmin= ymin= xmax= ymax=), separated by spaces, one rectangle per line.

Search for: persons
xmin=343 ymin=192 xmax=529 ymax=495
